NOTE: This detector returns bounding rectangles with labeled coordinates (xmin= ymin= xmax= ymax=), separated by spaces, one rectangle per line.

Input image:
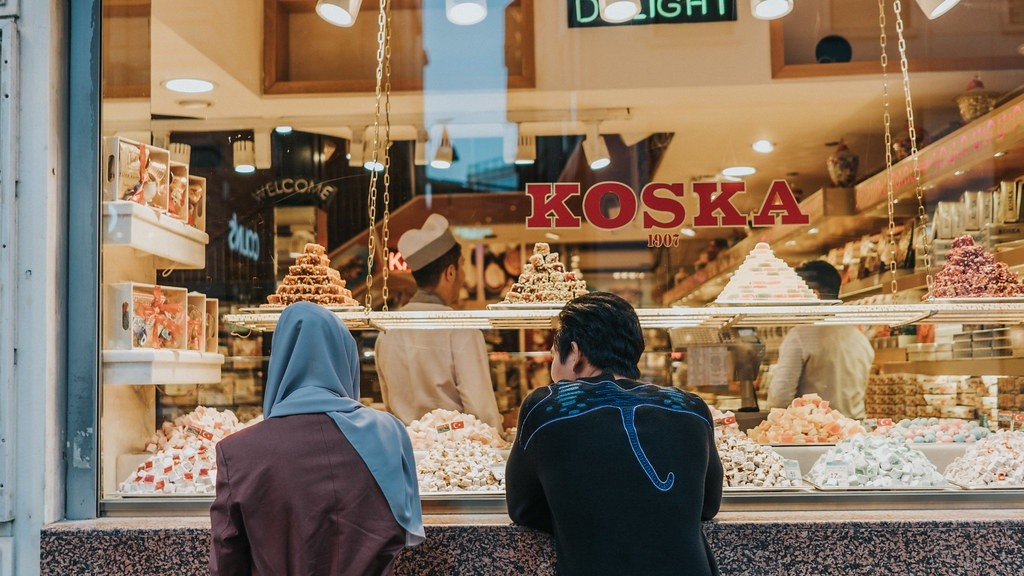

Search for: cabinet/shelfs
xmin=663 ymin=89 xmax=1024 ymax=433
xmin=100 ymin=136 xmax=225 ymax=386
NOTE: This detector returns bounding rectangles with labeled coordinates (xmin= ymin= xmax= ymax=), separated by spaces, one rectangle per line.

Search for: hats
xmin=397 ymin=213 xmax=456 ymax=272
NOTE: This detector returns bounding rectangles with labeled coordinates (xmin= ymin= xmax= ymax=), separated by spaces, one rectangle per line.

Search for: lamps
xmin=445 ymin=0 xmax=488 ymax=27
xmin=364 ymin=127 xmax=387 ymax=171
xmin=316 ymin=0 xmax=363 ymax=28
xmin=749 ymin=0 xmax=794 ymax=21
xmin=232 ymin=141 xmax=256 ymax=173
xmin=915 ymin=0 xmax=960 ymax=20
xmin=599 ymin=0 xmax=641 ymax=23
xmin=430 ymin=125 xmax=452 ymax=169
xmin=582 ymin=120 xmax=611 ymax=171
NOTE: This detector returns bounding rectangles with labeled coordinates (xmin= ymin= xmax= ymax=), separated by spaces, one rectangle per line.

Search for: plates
xmin=239 ymin=306 xmax=370 ymax=314
xmin=487 ymin=303 xmax=569 ymax=309
xmin=928 ymin=298 xmax=1024 ymax=302
xmin=714 ymin=299 xmax=844 ymax=307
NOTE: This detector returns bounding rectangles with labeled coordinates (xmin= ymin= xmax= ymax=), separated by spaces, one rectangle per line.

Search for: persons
xmin=765 ymin=261 xmax=876 ymax=422
xmin=504 ymin=291 xmax=725 ymax=576
xmin=371 ymin=214 xmax=508 ymax=438
xmin=207 ymin=302 xmax=427 ymax=576
